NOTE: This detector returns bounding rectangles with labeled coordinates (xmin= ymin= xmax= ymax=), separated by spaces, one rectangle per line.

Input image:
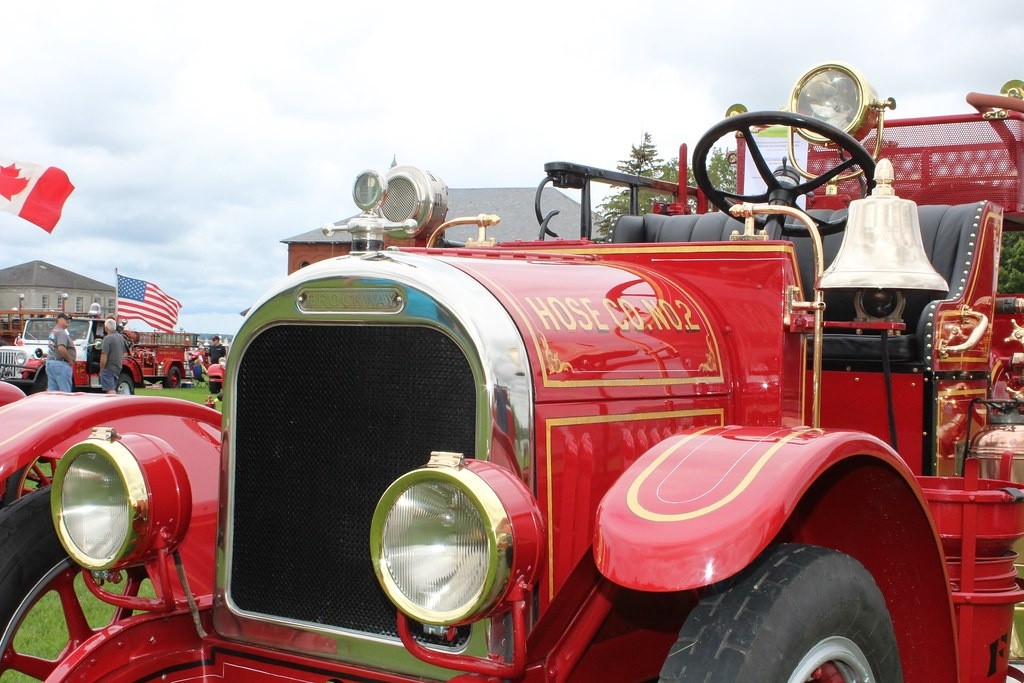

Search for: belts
xmin=50 ymin=358 xmax=67 ymax=361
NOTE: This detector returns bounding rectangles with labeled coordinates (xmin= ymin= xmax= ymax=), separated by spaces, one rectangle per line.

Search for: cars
xmin=0 ymin=59 xmax=1024 ymax=683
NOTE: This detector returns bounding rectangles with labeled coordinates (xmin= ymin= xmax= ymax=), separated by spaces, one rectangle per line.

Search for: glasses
xmin=213 ymin=339 xmax=215 ymax=341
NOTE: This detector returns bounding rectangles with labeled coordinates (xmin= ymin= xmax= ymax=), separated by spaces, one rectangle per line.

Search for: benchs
xmin=604 ymin=200 xmax=1003 ymax=476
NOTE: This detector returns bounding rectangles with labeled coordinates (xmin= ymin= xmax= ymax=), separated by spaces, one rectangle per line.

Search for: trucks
xmin=0 ymin=306 xmax=187 ymax=389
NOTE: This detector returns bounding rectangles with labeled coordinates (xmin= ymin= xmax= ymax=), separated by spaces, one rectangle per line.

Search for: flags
xmin=116 ymin=274 xmax=184 ymax=336
xmin=1 ymin=158 xmax=75 ymax=234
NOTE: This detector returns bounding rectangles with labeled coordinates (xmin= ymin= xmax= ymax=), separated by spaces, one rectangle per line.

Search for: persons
xmin=184 ymin=334 xmax=230 ymax=383
xmin=14 ymin=333 xmax=22 ymax=346
xmin=99 ymin=318 xmax=126 ymax=394
xmin=207 ymin=335 xmax=227 ymax=365
xmin=45 ymin=313 xmax=77 ymax=393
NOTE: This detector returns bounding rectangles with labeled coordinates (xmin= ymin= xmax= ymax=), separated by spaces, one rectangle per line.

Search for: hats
xmin=58 ymin=312 xmax=72 ymax=319
xmin=211 ymin=335 xmax=220 ymax=338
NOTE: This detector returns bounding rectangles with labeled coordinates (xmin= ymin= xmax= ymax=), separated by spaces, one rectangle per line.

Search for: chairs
xmin=192 ymin=365 xmax=206 ymax=389
